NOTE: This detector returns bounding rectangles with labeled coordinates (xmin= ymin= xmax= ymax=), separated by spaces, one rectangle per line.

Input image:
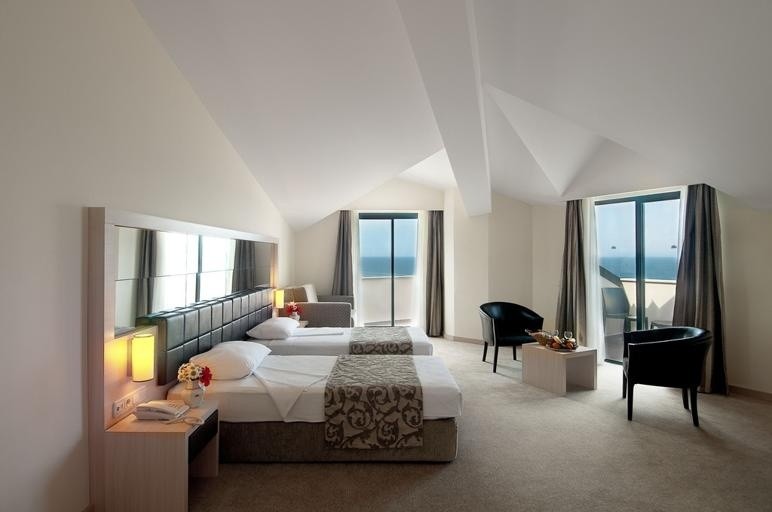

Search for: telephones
xmin=134 ymin=400 xmax=190 ymax=420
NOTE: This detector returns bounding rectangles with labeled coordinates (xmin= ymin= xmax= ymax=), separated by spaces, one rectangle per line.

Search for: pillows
xmin=246 ymin=317 xmax=301 ymax=340
xmin=189 ymin=341 xmax=272 ymax=381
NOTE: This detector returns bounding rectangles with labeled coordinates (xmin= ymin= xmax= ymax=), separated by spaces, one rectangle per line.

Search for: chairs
xmin=479 ymin=301 xmax=544 ymax=373
xmin=623 ymin=326 xmax=713 ymax=427
xmin=601 ymin=287 xmax=648 ymax=344
xmin=280 ymin=284 xmax=354 ymax=327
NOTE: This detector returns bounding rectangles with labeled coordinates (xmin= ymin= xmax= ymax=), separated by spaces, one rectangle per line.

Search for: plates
xmin=546 ymin=344 xmax=571 ymax=353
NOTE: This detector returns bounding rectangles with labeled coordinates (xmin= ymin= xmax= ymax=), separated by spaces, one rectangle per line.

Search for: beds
xmin=244 ymin=328 xmax=433 ymax=355
xmin=167 ymin=355 xmax=463 ymax=463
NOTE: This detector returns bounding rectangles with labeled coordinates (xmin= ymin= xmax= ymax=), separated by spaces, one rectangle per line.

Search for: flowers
xmin=178 ymin=362 xmax=212 ymax=386
xmin=287 ymin=301 xmax=303 ymax=313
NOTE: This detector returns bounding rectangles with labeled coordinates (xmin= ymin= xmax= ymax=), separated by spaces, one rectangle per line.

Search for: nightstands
xmin=105 ymin=400 xmax=220 ymax=512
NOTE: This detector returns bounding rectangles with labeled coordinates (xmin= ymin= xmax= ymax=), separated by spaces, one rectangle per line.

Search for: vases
xmin=289 ymin=312 xmax=300 ymax=321
xmin=183 ymin=381 xmax=205 ymax=408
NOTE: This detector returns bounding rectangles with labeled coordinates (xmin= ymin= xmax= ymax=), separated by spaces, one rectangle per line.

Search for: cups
xmin=551 ymin=329 xmax=558 ymax=336
xmin=564 ymin=331 xmax=572 ymax=340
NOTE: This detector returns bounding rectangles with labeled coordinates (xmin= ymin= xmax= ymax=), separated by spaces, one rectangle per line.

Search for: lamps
xmin=275 ymin=289 xmax=284 ymax=309
xmin=131 ymin=334 xmax=155 ymax=382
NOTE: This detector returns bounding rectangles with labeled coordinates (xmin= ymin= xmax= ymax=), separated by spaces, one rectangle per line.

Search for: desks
xmin=649 ymin=321 xmax=672 ymax=329
